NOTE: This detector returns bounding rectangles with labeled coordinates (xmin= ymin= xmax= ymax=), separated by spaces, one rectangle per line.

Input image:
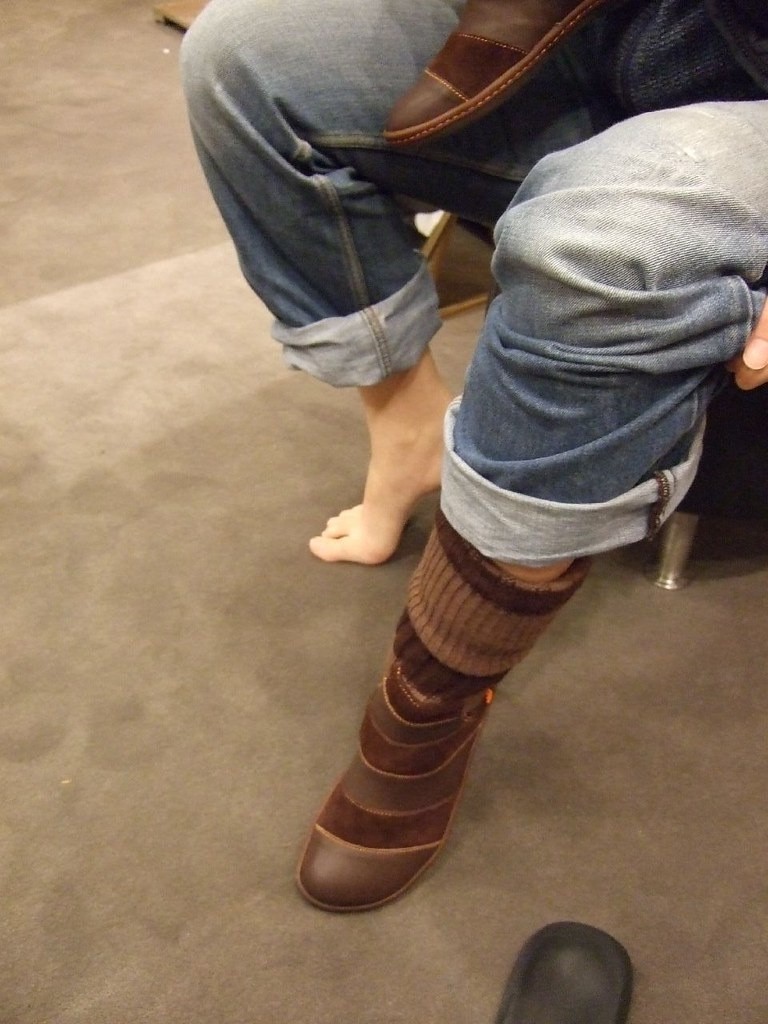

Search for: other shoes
xmin=494 ymin=921 xmax=633 ymax=1024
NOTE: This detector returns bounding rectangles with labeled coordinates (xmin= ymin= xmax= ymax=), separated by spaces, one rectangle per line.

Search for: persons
xmin=180 ymin=1 xmax=768 ymax=916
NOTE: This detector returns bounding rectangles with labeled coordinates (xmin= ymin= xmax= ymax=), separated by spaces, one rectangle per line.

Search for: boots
xmin=296 ymin=506 xmax=591 ymax=914
xmin=383 ymin=0 xmax=598 ymax=149
